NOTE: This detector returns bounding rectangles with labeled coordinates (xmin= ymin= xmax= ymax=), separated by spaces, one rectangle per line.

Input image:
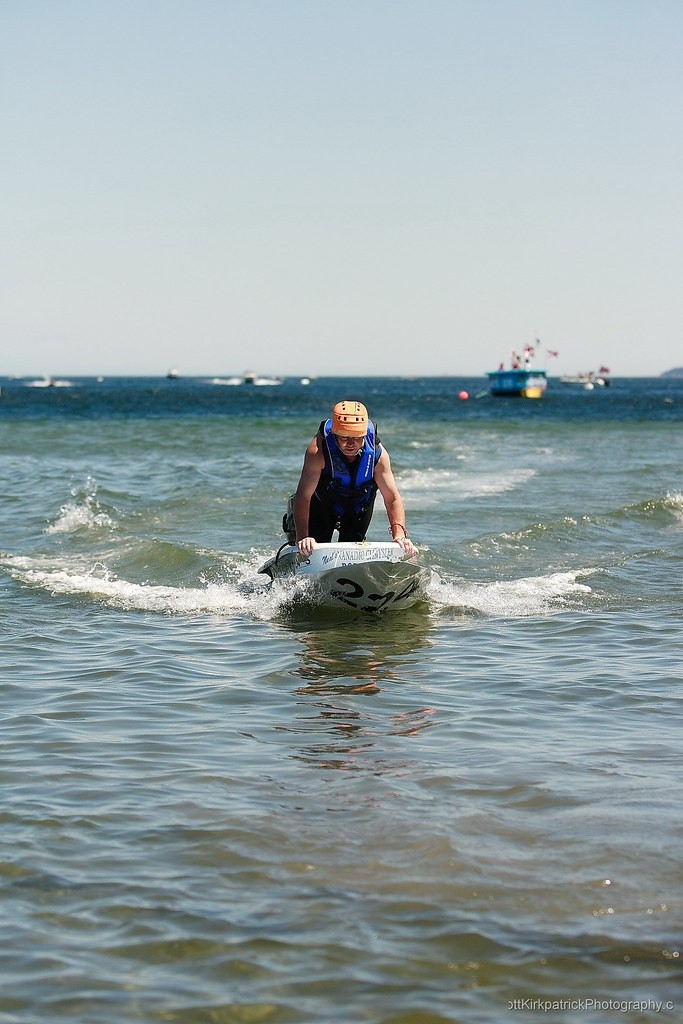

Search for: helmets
xmin=331 ymin=400 xmax=368 ymax=437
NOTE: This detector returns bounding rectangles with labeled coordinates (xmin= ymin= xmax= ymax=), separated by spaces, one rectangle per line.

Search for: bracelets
xmin=393 ymin=523 xmax=406 ymax=535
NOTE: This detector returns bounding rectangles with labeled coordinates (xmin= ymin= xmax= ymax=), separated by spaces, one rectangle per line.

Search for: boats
xmin=559 ymin=377 xmax=593 ymax=386
xmin=244 ymin=368 xmax=257 ymax=387
xmin=254 ymin=535 xmax=432 ymax=612
xmin=486 ymin=370 xmax=547 ymax=398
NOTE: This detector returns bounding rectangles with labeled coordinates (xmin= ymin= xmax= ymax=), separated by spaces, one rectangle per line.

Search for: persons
xmin=293 ymin=400 xmax=415 ymax=556
